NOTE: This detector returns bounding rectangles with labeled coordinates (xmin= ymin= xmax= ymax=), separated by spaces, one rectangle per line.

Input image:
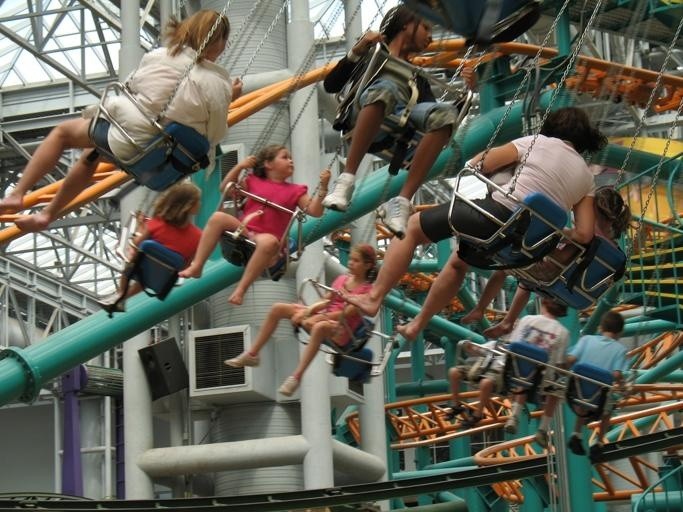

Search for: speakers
xmin=137 ymin=337 xmax=189 ymax=402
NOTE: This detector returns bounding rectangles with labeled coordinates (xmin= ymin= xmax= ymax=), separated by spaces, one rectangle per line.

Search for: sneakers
xmin=590 ymin=438 xmax=602 ymax=462
xmin=278 ymin=376 xmax=299 ymax=396
xmin=504 ymin=416 xmax=519 ymax=434
xmin=567 ymin=435 xmax=587 ymax=455
xmin=321 ymin=179 xmax=354 ymax=213
xmin=535 ymin=428 xmax=550 ymax=449
xmin=223 ymin=351 xmax=258 ymax=368
xmin=375 ymin=196 xmax=410 ymax=241
xmin=97 ymin=298 xmax=124 ymax=312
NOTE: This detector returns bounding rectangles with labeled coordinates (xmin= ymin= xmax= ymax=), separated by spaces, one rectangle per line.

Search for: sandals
xmin=444 ymin=406 xmax=464 ymax=421
xmin=462 ymin=409 xmax=484 ymax=426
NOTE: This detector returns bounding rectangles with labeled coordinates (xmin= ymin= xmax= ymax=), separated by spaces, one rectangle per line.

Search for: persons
xmin=0 ymin=8 xmax=242 ymax=232
xmin=179 ymin=149 xmax=330 ymax=305
xmin=327 ymin=9 xmax=460 ymax=236
xmin=226 ymin=242 xmax=376 ymax=392
xmin=460 ymin=189 xmax=630 ymax=337
xmin=101 ymin=182 xmax=208 ymax=312
xmin=445 ymin=296 xmax=624 ymax=457
xmin=341 ymin=108 xmax=609 ymax=339
